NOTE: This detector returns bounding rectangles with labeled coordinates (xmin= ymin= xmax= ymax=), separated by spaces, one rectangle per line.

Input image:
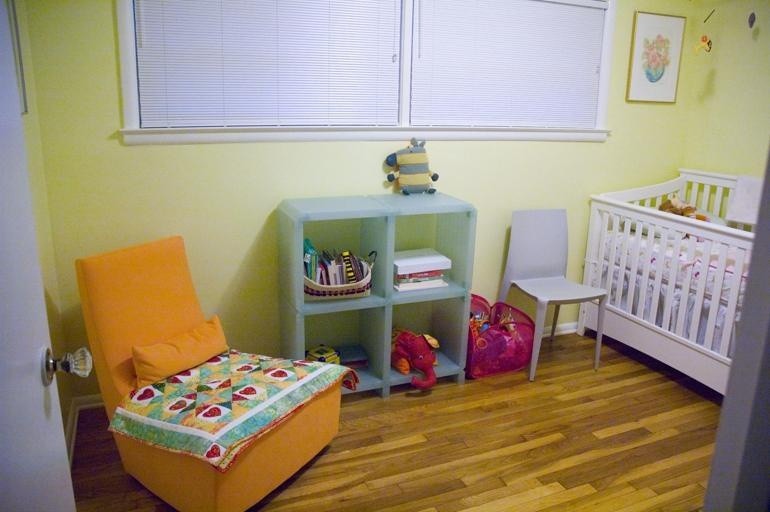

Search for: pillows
xmin=126 ymin=311 xmax=235 ymax=392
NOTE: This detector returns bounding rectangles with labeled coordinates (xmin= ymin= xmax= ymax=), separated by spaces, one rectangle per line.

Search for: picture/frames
xmin=624 ymin=9 xmax=688 ymax=105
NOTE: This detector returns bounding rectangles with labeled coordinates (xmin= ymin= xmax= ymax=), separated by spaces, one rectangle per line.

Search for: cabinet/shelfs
xmin=266 ymin=183 xmax=481 ymax=400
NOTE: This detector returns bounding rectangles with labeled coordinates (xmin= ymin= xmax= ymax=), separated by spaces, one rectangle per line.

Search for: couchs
xmin=72 ymin=232 xmax=361 ymax=512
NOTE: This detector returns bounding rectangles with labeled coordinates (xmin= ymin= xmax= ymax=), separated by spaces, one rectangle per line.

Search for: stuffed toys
xmin=391 ymin=327 xmax=440 ymax=389
xmin=384 ymin=138 xmax=439 ymax=195
xmin=657 ymin=197 xmax=708 ymax=223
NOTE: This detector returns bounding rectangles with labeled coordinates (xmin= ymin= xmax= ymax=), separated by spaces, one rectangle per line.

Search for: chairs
xmin=493 ymin=204 xmax=610 ymax=385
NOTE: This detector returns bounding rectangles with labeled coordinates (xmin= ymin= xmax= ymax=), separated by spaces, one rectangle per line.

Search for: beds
xmin=576 ymin=165 xmax=768 ymax=406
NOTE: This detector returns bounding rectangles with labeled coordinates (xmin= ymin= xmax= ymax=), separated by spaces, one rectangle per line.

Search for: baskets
xmin=303 ymin=250 xmax=377 ymax=301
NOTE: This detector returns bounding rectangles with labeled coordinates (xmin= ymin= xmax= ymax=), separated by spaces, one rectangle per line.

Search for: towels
xmin=723 ymin=169 xmax=766 ymax=226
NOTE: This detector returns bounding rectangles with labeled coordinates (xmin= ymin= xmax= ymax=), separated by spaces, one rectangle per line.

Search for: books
xmin=302 ymin=236 xmax=452 ymax=293
